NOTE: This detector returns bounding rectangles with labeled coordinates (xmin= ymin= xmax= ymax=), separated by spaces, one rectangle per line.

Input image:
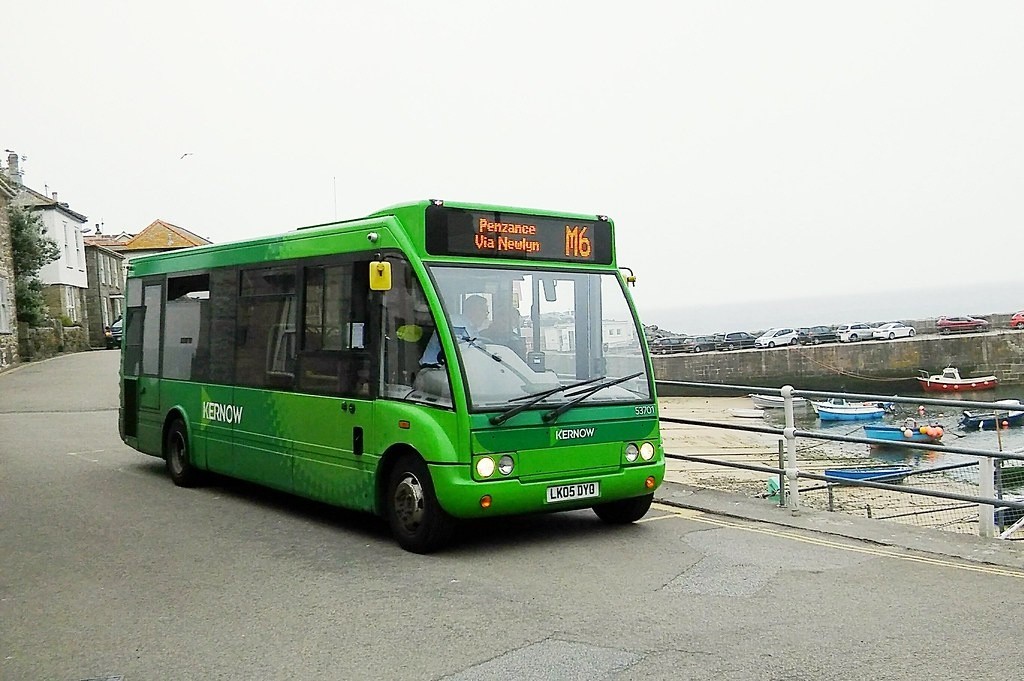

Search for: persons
xmin=479 ymin=304 xmax=526 ymax=363
xmin=415 ymin=295 xmax=489 ymax=376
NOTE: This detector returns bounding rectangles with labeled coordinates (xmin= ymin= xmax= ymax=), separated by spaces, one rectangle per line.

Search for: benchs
xmin=263 ymin=323 xmax=322 ymax=387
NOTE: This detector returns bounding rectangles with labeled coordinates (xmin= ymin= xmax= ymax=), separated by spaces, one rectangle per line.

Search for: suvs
xmin=683 ymin=335 xmax=716 ymax=353
xmin=714 ymin=332 xmax=758 ymax=353
xmin=836 ymin=323 xmax=878 ymax=342
xmin=650 ymin=338 xmax=684 ymax=355
xmin=629 ymin=340 xmax=654 ymax=356
xmin=935 ymin=314 xmax=988 ymax=335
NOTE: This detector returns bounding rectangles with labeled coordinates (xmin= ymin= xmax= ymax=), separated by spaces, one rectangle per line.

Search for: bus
xmin=117 ymin=199 xmax=664 ymax=549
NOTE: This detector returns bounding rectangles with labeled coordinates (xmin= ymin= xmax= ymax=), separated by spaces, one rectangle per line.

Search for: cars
xmin=1010 ymin=312 xmax=1024 ymax=329
xmin=753 ymin=328 xmax=798 ymax=348
xmin=798 ymin=325 xmax=838 ymax=345
xmin=104 ymin=317 xmax=122 ymax=348
xmin=873 ymin=323 xmax=917 ymax=340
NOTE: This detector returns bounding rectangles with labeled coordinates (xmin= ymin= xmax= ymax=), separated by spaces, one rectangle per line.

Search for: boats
xmin=993 ymin=497 xmax=1024 ymax=526
xmin=824 ymin=466 xmax=911 ymax=486
xmin=864 ymin=418 xmax=943 ymax=442
xmin=748 ymin=394 xmax=806 ymax=410
xmin=960 ymin=398 xmax=1024 ymax=429
xmin=808 ymin=399 xmax=894 ymax=415
xmin=729 ymin=408 xmax=766 ymax=418
xmin=816 ymin=406 xmax=885 ymax=421
xmin=917 ymin=367 xmax=997 ymax=389
xmin=992 ymin=448 xmax=1024 ymax=489
